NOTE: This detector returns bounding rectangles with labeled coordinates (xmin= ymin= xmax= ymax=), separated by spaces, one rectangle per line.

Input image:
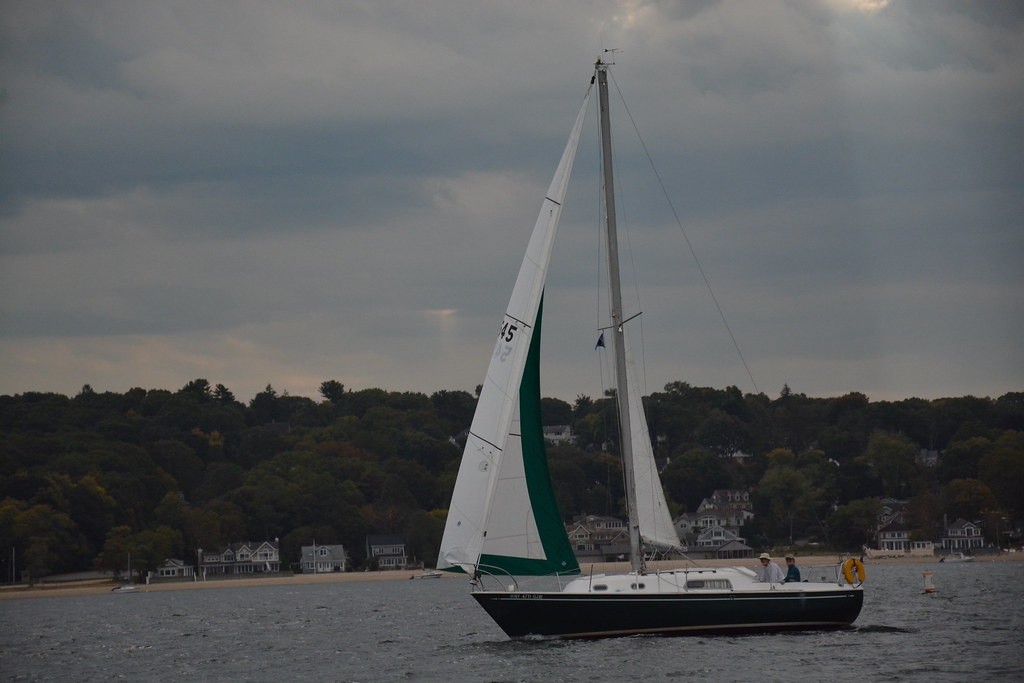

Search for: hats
xmin=758 ymin=553 xmax=772 ymax=559
xmin=784 ymin=553 xmax=795 ymax=559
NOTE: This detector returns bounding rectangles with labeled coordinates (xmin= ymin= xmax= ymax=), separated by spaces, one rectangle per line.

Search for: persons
xmin=779 ymin=554 xmax=800 ymax=585
xmin=758 ymin=553 xmax=785 ymax=584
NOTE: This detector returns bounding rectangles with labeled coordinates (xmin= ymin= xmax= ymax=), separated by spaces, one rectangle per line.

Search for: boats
xmin=939 ymin=552 xmax=975 ymax=563
xmin=113 ymin=586 xmax=140 ymax=593
xmin=409 ymin=571 xmax=443 ymax=580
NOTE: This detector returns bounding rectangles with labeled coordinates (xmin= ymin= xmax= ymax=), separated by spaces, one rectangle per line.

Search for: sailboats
xmin=436 ymin=57 xmax=864 ymax=642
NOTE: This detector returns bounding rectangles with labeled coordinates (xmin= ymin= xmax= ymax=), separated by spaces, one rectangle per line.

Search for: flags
xmin=594 ymin=333 xmax=606 ymax=351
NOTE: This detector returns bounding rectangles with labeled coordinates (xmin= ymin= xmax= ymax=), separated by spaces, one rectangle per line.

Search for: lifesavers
xmin=841 ymin=556 xmax=866 ymax=585
